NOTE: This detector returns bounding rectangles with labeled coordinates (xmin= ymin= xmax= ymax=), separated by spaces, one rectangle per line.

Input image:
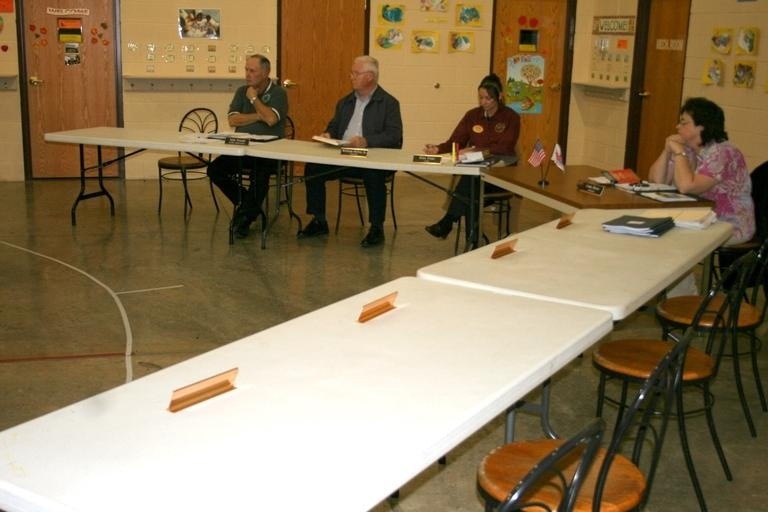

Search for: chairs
xmin=158 ymin=107 xmax=219 ymax=220
xmin=335 ymin=171 xmax=396 ymax=235
xmin=259 ymin=117 xmax=304 ymax=249
xmin=454 ymin=190 xmax=514 ymax=256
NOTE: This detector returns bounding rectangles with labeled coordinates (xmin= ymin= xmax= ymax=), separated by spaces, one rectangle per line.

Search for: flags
xmin=551 ymin=143 xmax=566 ymax=173
xmin=527 ymin=141 xmax=546 ymax=168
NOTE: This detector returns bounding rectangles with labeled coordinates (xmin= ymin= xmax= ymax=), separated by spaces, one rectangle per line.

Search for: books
xmin=312 ymin=135 xmax=352 ymax=146
xmin=615 ymin=181 xmax=699 ymax=204
xmin=207 ymin=131 xmax=280 ymax=142
xmin=600 ymin=214 xmax=675 ymax=239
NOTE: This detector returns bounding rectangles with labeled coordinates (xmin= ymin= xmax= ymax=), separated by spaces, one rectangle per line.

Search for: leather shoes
xmin=231 ymin=204 xmax=249 ymax=238
xmin=298 ymin=219 xmax=328 ymax=238
xmin=464 ymin=242 xmax=477 ymax=254
xmin=425 ymin=217 xmax=452 ymax=238
xmin=361 ymin=226 xmax=385 ymax=246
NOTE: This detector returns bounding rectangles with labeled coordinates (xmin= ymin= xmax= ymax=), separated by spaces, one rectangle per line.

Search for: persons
xmin=184 ymin=12 xmax=219 ymax=37
xmin=648 ymin=96 xmax=757 ymax=300
xmin=423 ymin=73 xmax=521 ymax=255
xmin=206 ymin=54 xmax=288 ymax=240
xmin=295 ymin=55 xmax=402 ymax=248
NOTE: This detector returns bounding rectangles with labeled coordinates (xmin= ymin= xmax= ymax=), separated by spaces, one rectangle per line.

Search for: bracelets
xmin=249 ymin=95 xmax=257 ymax=105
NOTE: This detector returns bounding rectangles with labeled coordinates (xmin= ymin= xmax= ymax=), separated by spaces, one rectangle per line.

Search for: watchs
xmin=672 ymin=152 xmax=689 ymax=158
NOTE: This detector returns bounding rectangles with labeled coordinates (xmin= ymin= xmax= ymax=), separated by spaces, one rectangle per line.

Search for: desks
xmin=477 ymin=162 xmax=713 ymax=235
xmin=243 ymin=139 xmax=497 ymax=256
xmin=43 ymin=124 xmax=245 ymax=238
